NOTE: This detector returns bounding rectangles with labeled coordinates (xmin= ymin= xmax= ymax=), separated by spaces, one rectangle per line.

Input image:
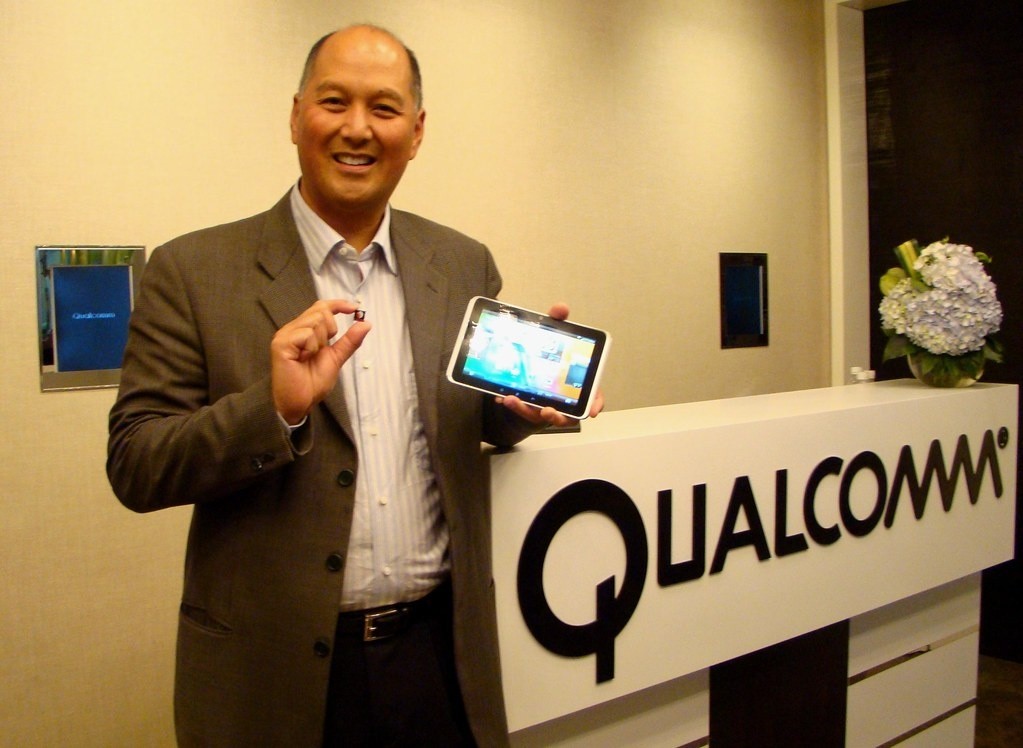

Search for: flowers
xmin=878 ymin=237 xmax=1003 ymax=362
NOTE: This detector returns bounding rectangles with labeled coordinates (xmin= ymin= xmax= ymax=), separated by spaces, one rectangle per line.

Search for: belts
xmin=334 ymin=589 xmax=445 ymax=646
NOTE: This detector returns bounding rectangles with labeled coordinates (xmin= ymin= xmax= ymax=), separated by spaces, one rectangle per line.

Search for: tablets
xmin=445 ymin=296 xmax=611 ymax=420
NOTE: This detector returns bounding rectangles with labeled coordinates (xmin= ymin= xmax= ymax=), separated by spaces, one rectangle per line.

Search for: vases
xmin=908 ymin=347 xmax=984 ymax=388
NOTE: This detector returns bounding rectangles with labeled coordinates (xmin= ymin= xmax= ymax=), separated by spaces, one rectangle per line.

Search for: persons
xmin=106 ymin=24 xmax=605 ymax=748
